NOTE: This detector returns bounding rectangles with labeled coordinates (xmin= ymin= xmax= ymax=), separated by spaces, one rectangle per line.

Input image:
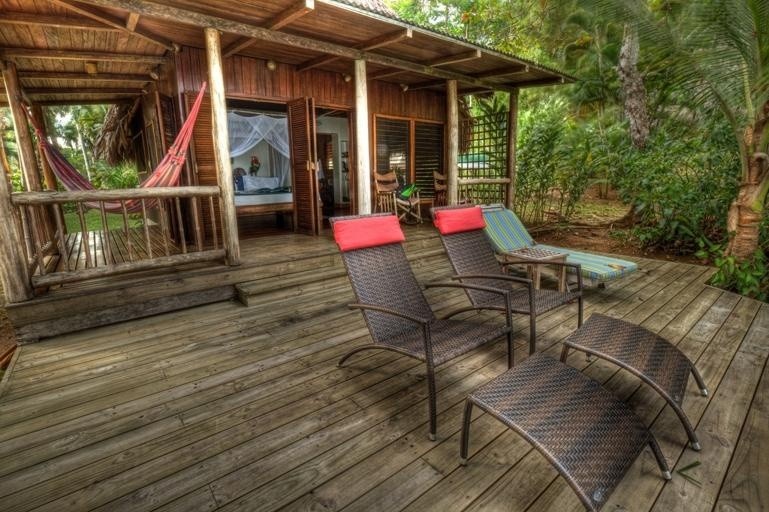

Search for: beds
xmin=228 ymin=113 xmax=294 ymax=232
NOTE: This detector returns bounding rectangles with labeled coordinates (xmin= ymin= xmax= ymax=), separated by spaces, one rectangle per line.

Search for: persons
xmin=319 ymin=178 xmax=335 ymax=225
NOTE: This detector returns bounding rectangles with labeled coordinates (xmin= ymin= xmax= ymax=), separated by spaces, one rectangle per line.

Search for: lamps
xmin=341 ymin=71 xmax=352 ymax=83
xmin=400 ymin=82 xmax=409 ymax=91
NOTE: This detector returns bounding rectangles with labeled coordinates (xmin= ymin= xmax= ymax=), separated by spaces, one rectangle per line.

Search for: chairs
xmin=482 ymin=201 xmax=646 ymax=296
xmin=373 ymin=169 xmax=422 ymax=227
xmin=322 ymin=202 xmax=519 ymax=441
xmin=429 ymin=198 xmax=587 ymax=360
xmin=433 ymin=170 xmax=473 ymax=205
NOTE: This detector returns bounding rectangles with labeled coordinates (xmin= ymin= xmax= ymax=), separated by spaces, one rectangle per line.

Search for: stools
xmin=557 ymin=309 xmax=714 ymax=456
xmin=457 ymin=347 xmax=675 ymax=511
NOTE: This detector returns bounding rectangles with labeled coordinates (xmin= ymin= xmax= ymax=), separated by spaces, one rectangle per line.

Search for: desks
xmin=418 ymin=195 xmax=435 ymax=224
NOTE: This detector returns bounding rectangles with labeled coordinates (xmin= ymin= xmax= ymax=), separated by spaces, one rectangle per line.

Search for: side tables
xmin=501 ymin=241 xmax=574 ymax=292
